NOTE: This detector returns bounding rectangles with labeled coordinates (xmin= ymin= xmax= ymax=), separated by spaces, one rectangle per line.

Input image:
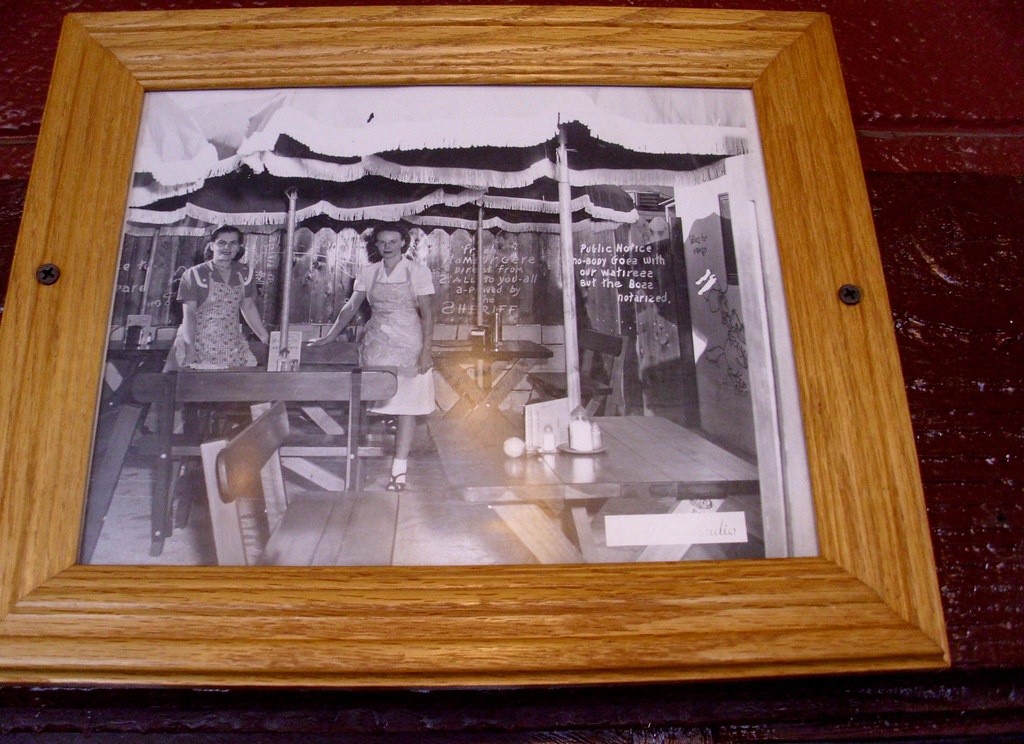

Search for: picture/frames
xmin=0 ymin=0 xmax=954 ymax=687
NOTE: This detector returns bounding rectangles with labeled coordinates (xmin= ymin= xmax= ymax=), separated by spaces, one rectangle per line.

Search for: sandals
xmin=385 ymin=471 xmax=408 ymax=492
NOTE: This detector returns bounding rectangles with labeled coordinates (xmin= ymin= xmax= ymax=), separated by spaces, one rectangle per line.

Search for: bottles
xmin=276 ymin=348 xmax=289 ymax=371
xmin=543 ymin=425 xmax=554 ymax=450
xmin=292 ymin=361 xmax=298 ymax=371
xmin=282 ymin=361 xmax=291 ymax=371
xmin=593 ymin=422 xmax=600 ymax=448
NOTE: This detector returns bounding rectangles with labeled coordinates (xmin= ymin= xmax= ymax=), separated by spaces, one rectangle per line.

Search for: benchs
xmin=132 ymin=371 xmax=398 ymax=557
xmin=725 ymin=446 xmax=764 ymax=543
xmin=200 ymin=401 xmax=400 ymax=566
xmin=527 ymin=330 xmax=622 ymax=416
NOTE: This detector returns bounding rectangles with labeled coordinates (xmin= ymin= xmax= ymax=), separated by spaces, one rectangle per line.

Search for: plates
xmin=559 ymin=444 xmax=607 ymax=454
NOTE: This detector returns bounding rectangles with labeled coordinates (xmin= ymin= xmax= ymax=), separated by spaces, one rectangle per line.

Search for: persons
xmin=161 ymin=225 xmax=269 ymax=373
xmin=306 ymin=222 xmax=436 ymax=490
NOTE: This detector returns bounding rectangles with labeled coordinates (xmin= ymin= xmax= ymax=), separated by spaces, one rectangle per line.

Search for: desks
xmin=424 ymin=413 xmax=761 ymax=564
xmin=100 ymin=340 xmax=554 ymax=435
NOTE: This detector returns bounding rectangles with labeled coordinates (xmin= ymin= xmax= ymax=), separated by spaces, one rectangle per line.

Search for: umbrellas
xmin=124 ymin=87 xmax=749 ymax=410
